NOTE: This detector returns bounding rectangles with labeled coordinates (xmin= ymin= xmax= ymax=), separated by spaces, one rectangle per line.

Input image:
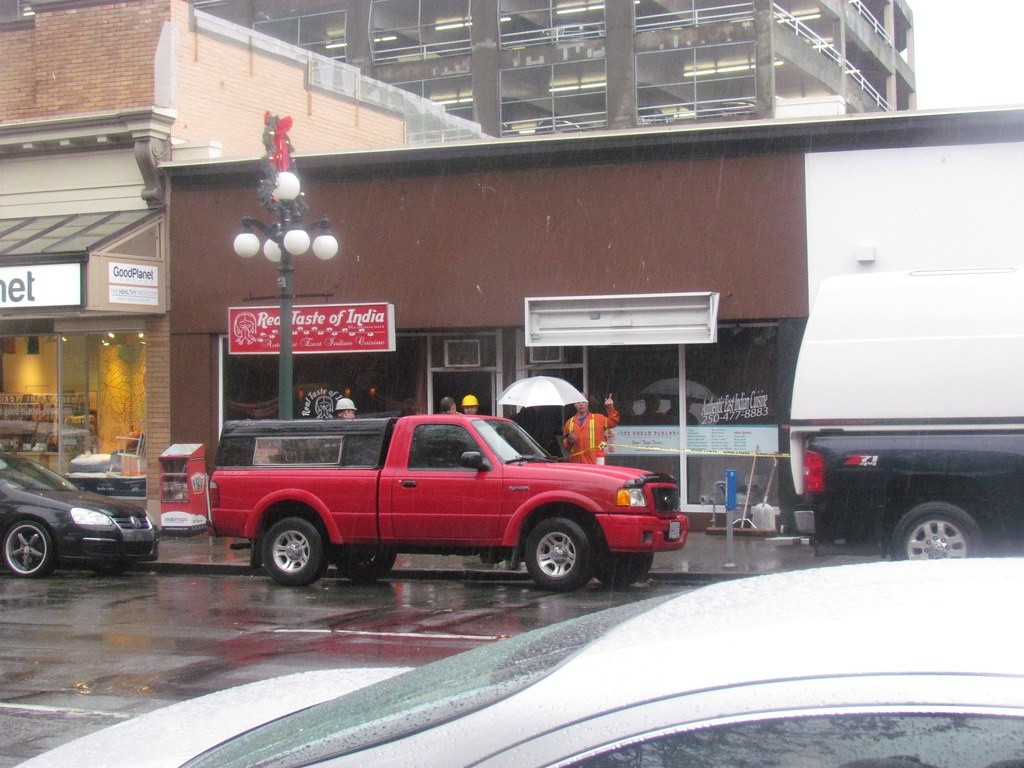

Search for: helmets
xmin=462 ymin=395 xmax=478 ymax=406
xmin=335 ymin=398 xmax=358 ymax=410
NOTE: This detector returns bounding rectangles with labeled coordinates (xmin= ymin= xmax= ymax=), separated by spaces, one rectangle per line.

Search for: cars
xmin=0 ymin=451 xmax=159 ymax=579
xmin=9 ymin=546 xmax=1024 ymax=767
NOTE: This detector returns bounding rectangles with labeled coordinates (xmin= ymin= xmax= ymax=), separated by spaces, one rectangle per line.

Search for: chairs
xmin=109 ymin=425 xmax=147 ymax=477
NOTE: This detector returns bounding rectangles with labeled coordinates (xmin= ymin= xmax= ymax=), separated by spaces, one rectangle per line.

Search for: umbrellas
xmin=495 ymin=376 xmax=588 ymax=408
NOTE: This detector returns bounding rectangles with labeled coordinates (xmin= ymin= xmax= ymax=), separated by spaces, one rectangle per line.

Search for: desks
xmin=17 ymin=452 xmax=70 ymax=476
xmin=0 ymin=420 xmax=90 ymax=455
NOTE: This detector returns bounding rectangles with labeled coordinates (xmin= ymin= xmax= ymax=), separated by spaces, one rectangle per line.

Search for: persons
xmin=514 ymin=406 xmax=564 ymax=454
xmin=335 ymin=398 xmax=358 ymax=418
xmin=440 ymin=397 xmax=456 ymax=414
xmin=563 ymin=393 xmax=619 ymax=464
xmin=461 ymin=395 xmax=479 ymax=415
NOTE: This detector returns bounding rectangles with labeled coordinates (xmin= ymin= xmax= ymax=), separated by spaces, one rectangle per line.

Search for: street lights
xmin=233 ymin=111 xmax=339 ymax=422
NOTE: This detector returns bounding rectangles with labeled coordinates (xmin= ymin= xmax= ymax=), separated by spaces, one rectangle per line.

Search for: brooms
xmin=205 ymin=476 xmax=215 ymax=536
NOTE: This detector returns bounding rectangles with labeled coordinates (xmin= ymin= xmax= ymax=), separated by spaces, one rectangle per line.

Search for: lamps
xmin=22 ymin=4 xmax=36 ymax=16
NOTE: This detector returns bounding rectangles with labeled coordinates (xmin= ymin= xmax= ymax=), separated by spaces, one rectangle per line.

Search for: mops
xmin=705 ymin=456 xmax=776 ymax=539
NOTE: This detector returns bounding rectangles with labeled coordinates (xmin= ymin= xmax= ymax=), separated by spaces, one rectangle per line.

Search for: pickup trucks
xmin=790 ymin=265 xmax=1024 ymax=560
xmin=207 ymin=412 xmax=692 ymax=586
